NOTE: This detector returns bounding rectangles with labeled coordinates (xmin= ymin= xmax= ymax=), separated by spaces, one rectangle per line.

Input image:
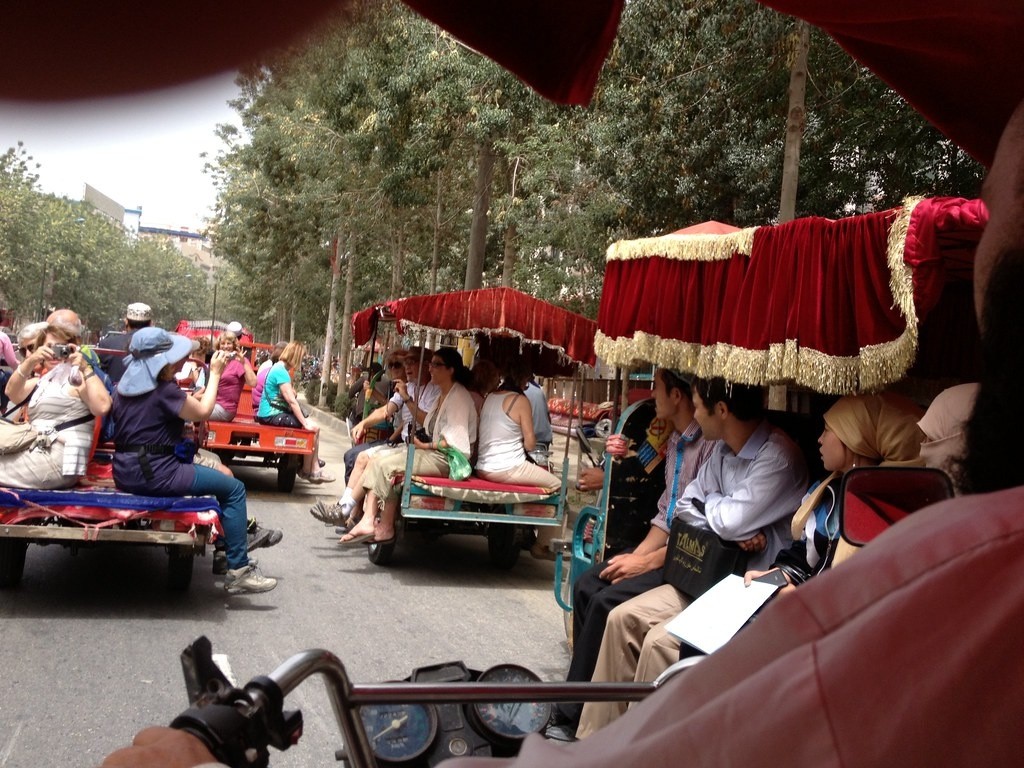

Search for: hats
xmin=127 ymin=302 xmax=152 ymax=322
xmin=401 ymin=351 xmax=433 ymax=360
xmin=117 ymin=327 xmax=200 ymax=397
xmin=669 ymin=368 xmax=694 ymax=384
xmin=227 ymin=321 xmax=242 ymax=337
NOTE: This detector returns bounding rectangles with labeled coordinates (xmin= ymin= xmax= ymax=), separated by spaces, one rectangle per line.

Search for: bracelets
xmin=84 ymin=371 xmax=95 ymax=380
xmin=16 ymin=364 xmax=32 ymax=379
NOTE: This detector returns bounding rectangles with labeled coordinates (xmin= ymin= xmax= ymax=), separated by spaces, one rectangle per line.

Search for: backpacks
xmin=80 ymin=349 xmax=115 ymax=442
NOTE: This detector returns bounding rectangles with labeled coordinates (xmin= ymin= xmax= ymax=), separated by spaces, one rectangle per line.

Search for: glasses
xmin=525 ymin=379 xmax=529 ymax=390
xmin=429 ymin=361 xmax=446 ymax=368
xmin=19 ymin=344 xmax=36 ymax=357
xmin=386 ymin=362 xmax=403 ymax=370
xmin=405 ymin=358 xmax=419 ymax=365
xmin=495 ymin=377 xmax=502 ymax=381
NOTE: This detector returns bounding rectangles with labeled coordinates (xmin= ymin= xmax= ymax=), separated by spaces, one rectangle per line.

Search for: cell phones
xmin=751 ymin=568 xmax=789 ymax=591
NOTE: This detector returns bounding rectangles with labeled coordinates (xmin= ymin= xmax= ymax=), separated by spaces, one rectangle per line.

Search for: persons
xmin=0 ymin=102 xmax=1022 ymax=768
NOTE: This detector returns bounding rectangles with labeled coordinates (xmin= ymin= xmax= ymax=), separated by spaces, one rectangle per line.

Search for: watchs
xmin=430 ymin=442 xmax=433 ymax=449
xmin=404 ymin=396 xmax=413 ymax=404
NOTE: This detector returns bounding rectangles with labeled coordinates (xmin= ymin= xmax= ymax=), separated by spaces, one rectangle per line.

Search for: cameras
xmin=229 ymin=353 xmax=235 ymax=360
xmin=406 ymin=433 xmax=430 ymax=445
xmin=51 ymin=344 xmax=72 ymax=358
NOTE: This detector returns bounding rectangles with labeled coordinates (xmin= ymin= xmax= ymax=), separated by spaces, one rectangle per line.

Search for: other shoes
xmin=517 ymin=532 xmax=570 ymax=562
xmin=310 ymin=501 xmax=360 ymax=534
xmin=318 ymin=459 xmax=325 ymax=467
xmin=546 ymin=725 xmax=575 ymax=742
xmin=299 ymin=469 xmax=336 ymax=484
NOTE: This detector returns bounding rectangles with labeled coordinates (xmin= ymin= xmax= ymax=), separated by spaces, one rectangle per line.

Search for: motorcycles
xmin=0 ymin=195 xmax=1024 ymax=686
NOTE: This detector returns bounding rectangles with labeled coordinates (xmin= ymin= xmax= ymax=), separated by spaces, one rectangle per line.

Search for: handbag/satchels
xmin=662 ymin=518 xmax=747 ymax=600
xmin=0 ymin=417 xmax=43 ymax=454
xmin=385 ymin=433 xmax=403 ymax=448
xmin=0 ymin=369 xmax=13 ymax=406
xmin=288 ymin=399 xmax=309 ymax=418
xmin=437 ymin=437 xmax=472 ymax=481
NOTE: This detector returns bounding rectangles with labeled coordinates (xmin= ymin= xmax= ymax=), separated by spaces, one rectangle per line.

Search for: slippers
xmin=338 ymin=532 xmax=395 ymax=545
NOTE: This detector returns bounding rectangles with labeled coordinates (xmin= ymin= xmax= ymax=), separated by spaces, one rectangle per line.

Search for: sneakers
xmin=213 ymin=515 xmax=283 ymax=594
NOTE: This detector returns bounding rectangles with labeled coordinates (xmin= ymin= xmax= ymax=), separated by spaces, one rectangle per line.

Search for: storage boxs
xmin=663 ymin=519 xmax=752 ymax=600
